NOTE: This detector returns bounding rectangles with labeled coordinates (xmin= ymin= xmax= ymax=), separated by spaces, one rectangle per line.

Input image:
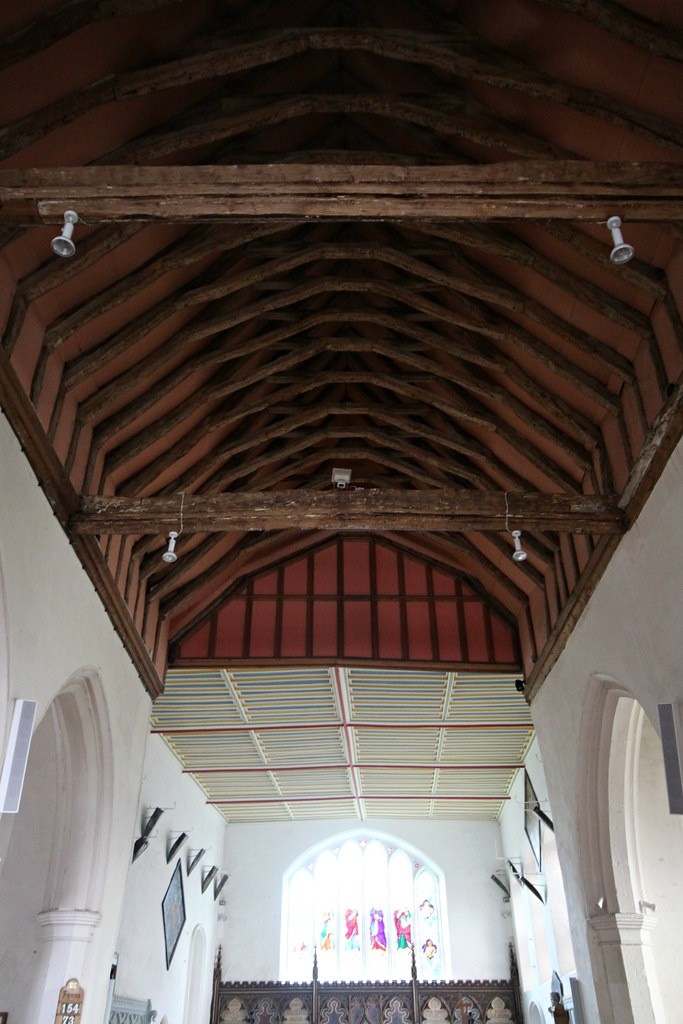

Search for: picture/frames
xmin=521 ymin=767 xmax=543 ymax=874
xmin=159 ymin=856 xmax=189 ymax=973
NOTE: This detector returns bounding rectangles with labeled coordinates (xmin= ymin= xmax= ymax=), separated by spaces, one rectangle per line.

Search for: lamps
xmin=48 ymin=207 xmax=83 ymax=261
xmin=594 ymin=895 xmax=605 ymax=911
xmin=158 ymin=529 xmax=181 ymax=565
xmin=508 ymin=527 xmax=529 ymax=563
xmin=605 ymin=211 xmax=638 ymax=268
xmin=641 ymin=900 xmax=658 ymax=913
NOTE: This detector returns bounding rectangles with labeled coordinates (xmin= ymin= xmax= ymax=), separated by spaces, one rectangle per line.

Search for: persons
xmin=549 ymin=992 xmax=569 ymax=1024
